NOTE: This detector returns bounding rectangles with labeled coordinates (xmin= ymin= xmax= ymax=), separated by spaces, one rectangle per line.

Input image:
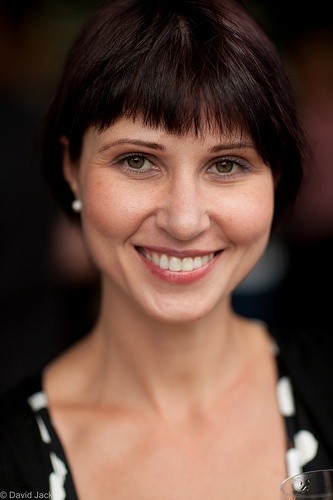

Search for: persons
xmin=0 ymin=0 xmax=332 ymax=499
